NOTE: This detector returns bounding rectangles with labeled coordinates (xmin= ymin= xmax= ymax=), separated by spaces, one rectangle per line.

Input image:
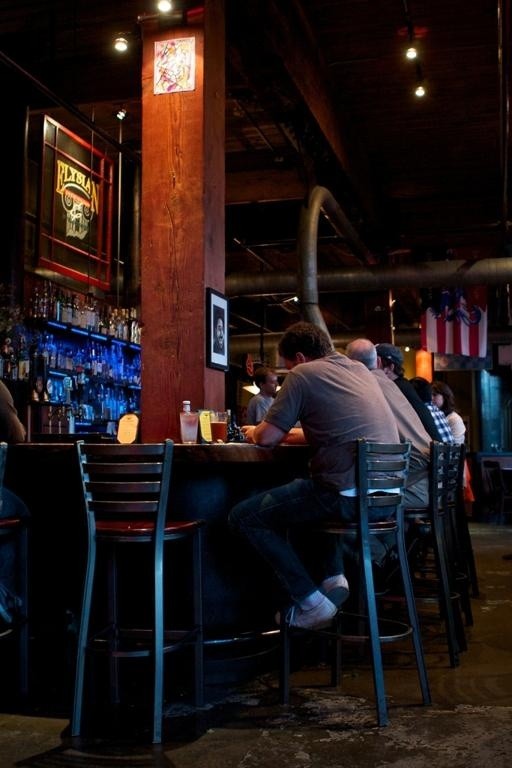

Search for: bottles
xmin=3 ymin=280 xmax=141 ymax=441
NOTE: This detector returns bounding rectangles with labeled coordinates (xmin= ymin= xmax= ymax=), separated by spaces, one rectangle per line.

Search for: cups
xmin=210 ymin=411 xmax=230 ymax=444
xmin=178 ymin=413 xmax=198 ymax=445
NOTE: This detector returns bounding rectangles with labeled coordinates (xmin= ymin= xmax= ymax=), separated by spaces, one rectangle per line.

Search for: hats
xmin=376 ymin=341 xmax=405 ymax=368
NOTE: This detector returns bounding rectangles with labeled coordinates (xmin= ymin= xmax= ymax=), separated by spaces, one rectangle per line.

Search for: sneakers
xmin=285 ymin=597 xmax=339 ymax=627
xmin=322 ymin=575 xmax=352 ymax=608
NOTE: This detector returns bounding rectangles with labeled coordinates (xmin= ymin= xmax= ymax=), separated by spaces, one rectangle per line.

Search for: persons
xmin=248 ymin=368 xmax=279 ymax=426
xmin=231 ymin=323 xmax=402 ymax=628
xmin=214 ymin=317 xmax=225 ymax=356
xmin=0 ymin=379 xmax=27 ymax=624
xmin=337 ymin=337 xmax=475 ymax=569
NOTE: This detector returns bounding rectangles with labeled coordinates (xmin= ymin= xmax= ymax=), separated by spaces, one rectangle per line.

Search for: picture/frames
xmin=206 ymin=286 xmax=232 ymax=372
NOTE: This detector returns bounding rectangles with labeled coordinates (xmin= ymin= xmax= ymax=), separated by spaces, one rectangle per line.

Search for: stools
xmin=396 ymin=444 xmax=479 ymax=660
xmin=71 ymin=437 xmax=206 ymax=745
xmin=273 ymin=438 xmax=431 ymax=718
